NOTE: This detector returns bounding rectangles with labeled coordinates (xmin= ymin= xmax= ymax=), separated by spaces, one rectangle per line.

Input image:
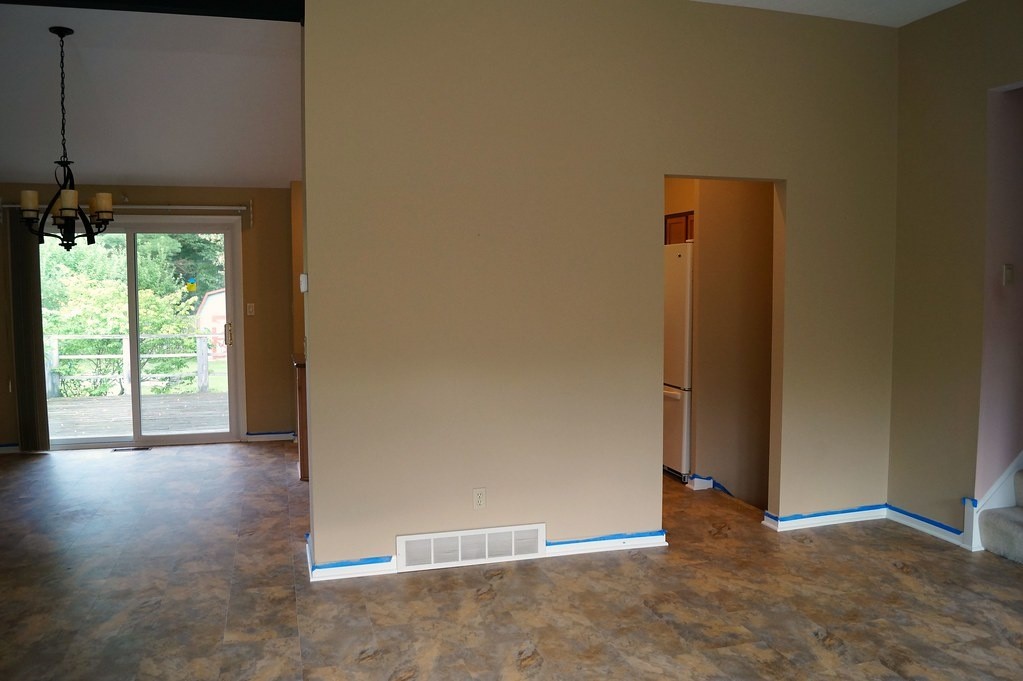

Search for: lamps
xmin=20 ymin=25 xmax=115 ymax=252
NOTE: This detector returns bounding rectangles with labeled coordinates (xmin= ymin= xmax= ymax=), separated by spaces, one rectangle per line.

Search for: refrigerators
xmin=662 ymin=239 xmax=695 ymax=485
